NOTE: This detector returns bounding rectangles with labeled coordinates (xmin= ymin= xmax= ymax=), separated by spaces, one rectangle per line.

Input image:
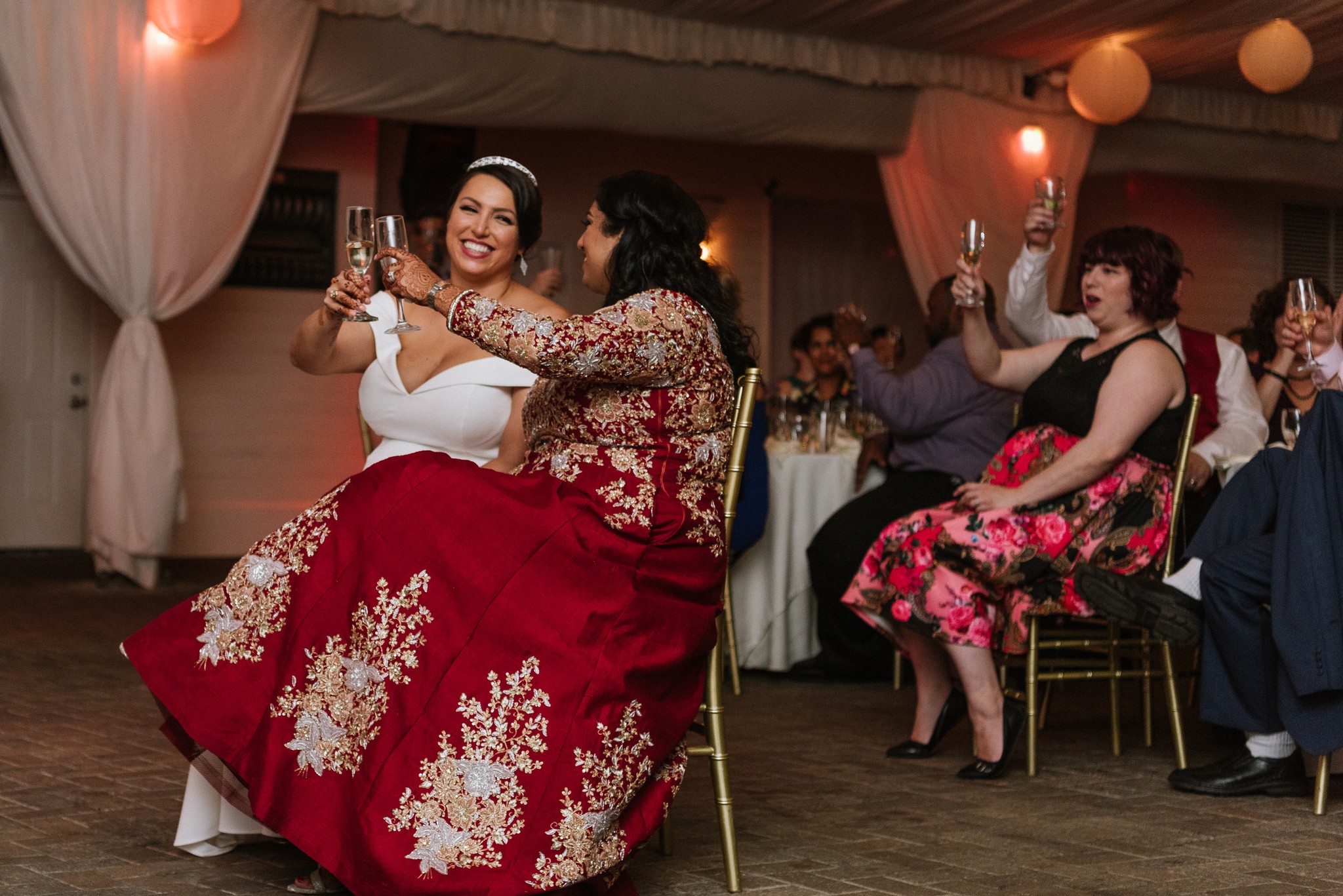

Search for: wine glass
xmin=1034 ymin=175 xmax=1068 ymax=230
xmin=1290 ymin=278 xmax=1328 ymax=371
xmin=955 ymin=218 xmax=986 ymax=307
xmin=341 ymin=206 xmax=379 ymax=322
xmin=375 ymin=215 xmax=422 ymax=334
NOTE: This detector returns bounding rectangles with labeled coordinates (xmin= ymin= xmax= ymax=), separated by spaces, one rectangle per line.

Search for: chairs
xmin=658 ymin=367 xmax=761 ymax=892
xmin=994 ymin=393 xmax=1203 ymax=773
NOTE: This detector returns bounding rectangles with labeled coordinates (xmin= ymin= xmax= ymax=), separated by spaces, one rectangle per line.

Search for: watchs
xmin=844 ymin=339 xmax=871 ymax=358
xmin=427 ymin=278 xmax=452 ymax=312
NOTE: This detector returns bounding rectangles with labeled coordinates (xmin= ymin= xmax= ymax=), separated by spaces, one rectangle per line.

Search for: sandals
xmin=286 ymin=862 xmax=348 ymax=894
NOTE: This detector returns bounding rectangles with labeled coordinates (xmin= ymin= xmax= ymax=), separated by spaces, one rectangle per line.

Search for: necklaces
xmin=1284 ymin=369 xmax=1319 ymax=402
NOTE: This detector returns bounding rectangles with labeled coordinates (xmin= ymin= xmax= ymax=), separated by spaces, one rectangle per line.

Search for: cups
xmin=774 ymin=394 xmax=852 ymax=453
xmin=1280 ymin=408 xmax=1304 ymax=446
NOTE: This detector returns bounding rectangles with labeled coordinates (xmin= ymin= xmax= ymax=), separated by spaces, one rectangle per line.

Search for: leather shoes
xmin=1169 ymin=744 xmax=1309 ymax=796
xmin=957 ymin=695 xmax=1027 ymax=780
xmin=886 ymin=685 xmax=967 ymax=761
xmin=788 ymin=651 xmax=894 ymax=685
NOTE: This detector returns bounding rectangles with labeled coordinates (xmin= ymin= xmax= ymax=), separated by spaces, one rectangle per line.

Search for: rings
xmin=386 ymin=270 xmax=396 ymax=283
xmin=329 ymin=289 xmax=340 ymax=300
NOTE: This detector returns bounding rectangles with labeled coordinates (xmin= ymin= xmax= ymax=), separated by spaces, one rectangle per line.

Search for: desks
xmin=728 ymin=424 xmax=886 ymax=671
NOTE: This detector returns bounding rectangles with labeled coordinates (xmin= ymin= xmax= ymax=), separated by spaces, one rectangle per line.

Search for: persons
xmin=1224 ymin=328 xmax=1263 ymax=384
xmin=705 ymin=271 xmax=770 ymax=576
xmin=430 ymin=222 xmax=565 ymax=305
xmin=115 ymin=172 xmax=749 ymax=896
xmin=1165 ymin=291 xmax=1343 ymax=800
xmin=841 ymin=225 xmax=1190 ymax=782
xmin=1231 ymin=272 xmax=1343 ymax=452
xmin=773 ymin=312 xmax=867 ymax=444
xmin=789 ymin=272 xmax=1018 ymax=684
xmin=1003 ymin=198 xmax=1268 ymax=572
xmin=855 ymin=325 xmax=906 ymax=441
xmin=173 ymin=151 xmax=575 ymax=874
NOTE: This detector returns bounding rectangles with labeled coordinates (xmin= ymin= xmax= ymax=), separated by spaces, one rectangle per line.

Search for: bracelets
xmin=1263 ymin=368 xmax=1287 ymax=384
